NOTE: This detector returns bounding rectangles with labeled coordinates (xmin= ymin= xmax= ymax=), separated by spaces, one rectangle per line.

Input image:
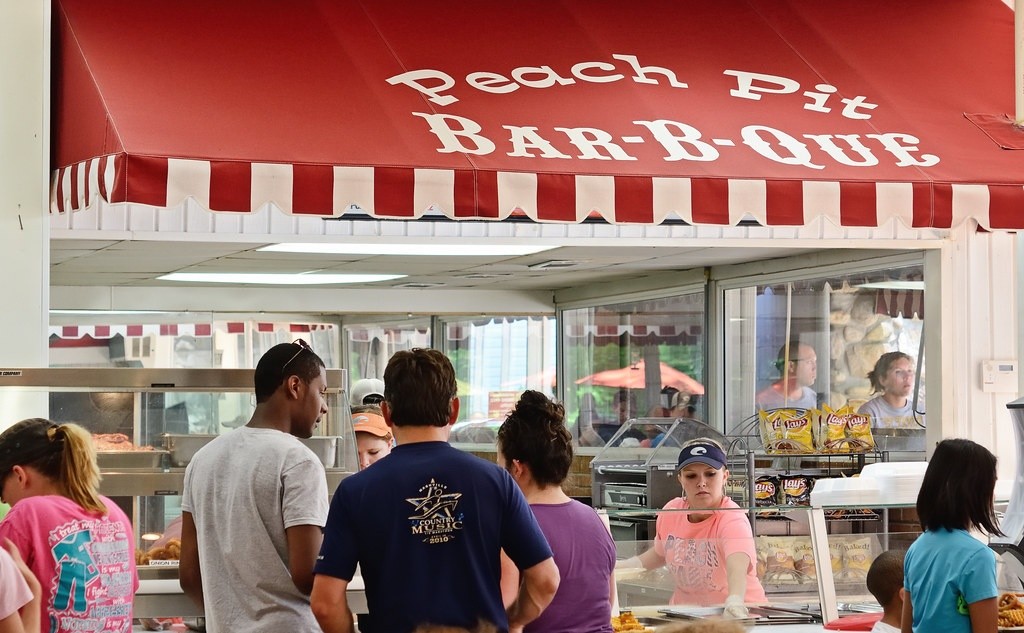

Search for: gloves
xmin=723 ymin=594 xmax=749 ymax=618
xmin=614 ymin=556 xmax=643 ymax=581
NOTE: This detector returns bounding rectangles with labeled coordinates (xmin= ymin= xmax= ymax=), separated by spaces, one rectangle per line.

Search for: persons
xmin=614 ymin=438 xmax=768 ymax=619
xmin=499 ymin=390 xmax=616 ymax=633
xmin=0 ymin=537 xmax=43 ymax=633
xmin=0 ymin=419 xmax=139 ymax=633
xmin=310 ymin=347 xmax=561 ymax=633
xmin=573 ymin=390 xmax=698 ymax=447
xmin=179 ymin=339 xmax=330 ymax=633
xmin=867 ymin=549 xmax=908 ymax=633
xmin=755 ymin=341 xmax=818 ymax=421
xmin=856 ymin=352 xmax=926 ymax=429
xmin=901 ymin=439 xmax=1007 ymax=633
xmin=347 ymin=378 xmax=395 ymax=477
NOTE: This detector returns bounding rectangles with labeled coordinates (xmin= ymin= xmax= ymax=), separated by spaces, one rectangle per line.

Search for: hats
xmin=346 ymin=413 xmax=393 ymax=437
xmin=677 ymin=443 xmax=728 ymax=471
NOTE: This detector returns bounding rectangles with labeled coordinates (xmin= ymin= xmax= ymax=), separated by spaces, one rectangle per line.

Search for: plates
xmin=997 ymin=626 xmax=1024 ymax=630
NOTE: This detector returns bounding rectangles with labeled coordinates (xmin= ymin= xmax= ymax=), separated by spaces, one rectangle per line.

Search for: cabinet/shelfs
xmin=725 ymin=407 xmax=890 ymax=567
xmin=0 ymin=367 xmax=369 ymax=633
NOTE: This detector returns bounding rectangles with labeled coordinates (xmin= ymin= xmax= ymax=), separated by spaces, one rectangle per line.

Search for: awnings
xmin=51 ymin=0 xmax=1024 ymax=232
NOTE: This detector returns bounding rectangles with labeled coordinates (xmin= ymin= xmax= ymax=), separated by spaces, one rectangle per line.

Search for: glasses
xmin=282 ymin=337 xmax=314 ymax=371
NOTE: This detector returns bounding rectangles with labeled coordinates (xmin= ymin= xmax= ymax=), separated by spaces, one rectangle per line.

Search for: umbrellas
xmin=575 ymin=358 xmax=705 ymax=396
